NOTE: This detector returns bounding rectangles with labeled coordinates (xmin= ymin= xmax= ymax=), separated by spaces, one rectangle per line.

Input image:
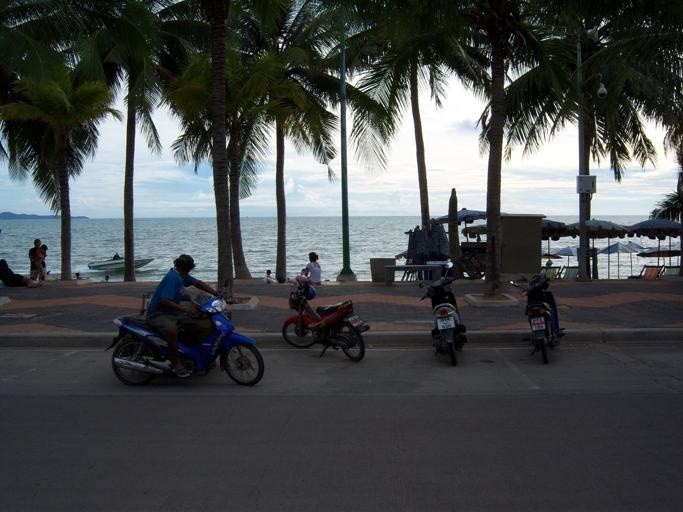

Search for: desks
xmin=382 ymin=264 xmax=442 ymax=286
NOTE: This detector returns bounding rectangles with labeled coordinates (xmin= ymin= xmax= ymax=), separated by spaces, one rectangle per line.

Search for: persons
xmin=147 ymin=254 xmax=222 ymax=379
xmin=113 ymin=253 xmax=120 ymax=260
xmin=266 ymin=270 xmax=273 ymax=283
xmin=29 ymin=239 xmax=45 ymax=280
xmin=0 ymin=259 xmax=40 ymax=287
xmin=76 ymin=273 xmax=81 ymax=280
xmin=104 ymin=272 xmax=110 ymax=280
xmin=287 ymin=252 xmax=321 ymax=285
xmin=35 ymin=244 xmax=48 ymax=281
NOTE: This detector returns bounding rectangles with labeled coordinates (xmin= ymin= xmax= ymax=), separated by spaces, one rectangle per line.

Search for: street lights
xmin=577 ymin=26 xmax=608 ymax=282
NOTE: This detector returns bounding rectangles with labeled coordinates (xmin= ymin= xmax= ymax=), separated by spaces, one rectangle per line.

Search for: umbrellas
xmin=397 ymin=207 xmax=487 ymax=282
xmin=542 ymin=217 xmax=682 ymax=279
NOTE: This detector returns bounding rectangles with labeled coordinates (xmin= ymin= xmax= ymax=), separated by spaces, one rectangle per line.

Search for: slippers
xmin=170 ymin=368 xmax=190 ymax=378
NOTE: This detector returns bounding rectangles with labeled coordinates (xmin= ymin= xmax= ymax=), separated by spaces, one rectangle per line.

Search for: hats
xmin=174 ymin=254 xmax=195 ymax=268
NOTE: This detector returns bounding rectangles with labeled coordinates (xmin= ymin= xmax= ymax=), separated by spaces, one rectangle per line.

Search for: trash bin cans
xmin=370 ymin=258 xmax=396 ymax=281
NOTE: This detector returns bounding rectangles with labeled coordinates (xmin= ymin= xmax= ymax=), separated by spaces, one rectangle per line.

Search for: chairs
xmin=539 ymin=265 xmax=579 ymax=281
xmin=627 ymin=265 xmax=679 ymax=280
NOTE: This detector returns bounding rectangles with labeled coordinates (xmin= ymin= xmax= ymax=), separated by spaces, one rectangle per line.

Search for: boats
xmin=87 ymin=257 xmax=155 ymax=272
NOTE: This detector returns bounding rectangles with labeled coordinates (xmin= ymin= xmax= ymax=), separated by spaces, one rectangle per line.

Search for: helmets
xmin=304 ymin=286 xmax=317 ymax=300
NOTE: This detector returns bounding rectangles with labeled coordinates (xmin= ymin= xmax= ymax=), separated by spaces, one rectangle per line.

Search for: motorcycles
xmin=282 ymin=268 xmax=370 ymax=362
xmin=418 ymin=261 xmax=469 ymax=367
xmin=104 ymin=277 xmax=265 ymax=387
xmin=509 ymin=260 xmax=566 ymax=365
